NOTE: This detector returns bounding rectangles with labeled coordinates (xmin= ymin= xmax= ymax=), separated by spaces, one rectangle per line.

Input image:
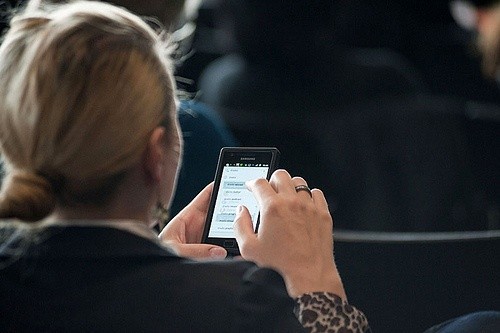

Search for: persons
xmin=0 ymin=0 xmax=499 ymax=333
xmin=100 ymin=0 xmax=233 ymax=232
xmin=201 ymin=0 xmax=489 ymax=118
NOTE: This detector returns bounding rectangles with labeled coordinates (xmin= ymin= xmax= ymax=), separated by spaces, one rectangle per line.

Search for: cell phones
xmin=200 ymin=147 xmax=281 ymax=257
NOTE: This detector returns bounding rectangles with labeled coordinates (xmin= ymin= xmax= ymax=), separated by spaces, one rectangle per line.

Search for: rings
xmin=295 ymin=184 xmax=313 ymax=196
